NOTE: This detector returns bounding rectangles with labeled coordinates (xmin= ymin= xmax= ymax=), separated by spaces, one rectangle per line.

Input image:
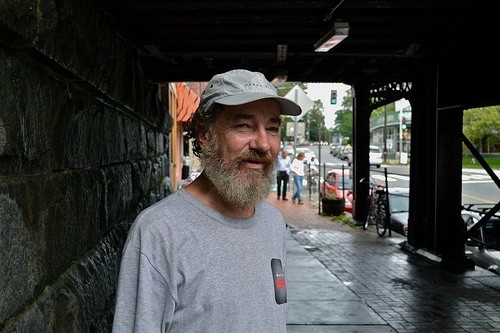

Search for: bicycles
xmin=362 ymin=182 xmax=389 ymax=237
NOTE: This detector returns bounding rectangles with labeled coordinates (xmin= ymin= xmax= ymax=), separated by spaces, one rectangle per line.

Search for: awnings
xmin=175 ymin=81 xmax=202 ymax=123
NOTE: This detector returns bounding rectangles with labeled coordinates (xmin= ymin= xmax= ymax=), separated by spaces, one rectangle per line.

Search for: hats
xmin=199 ymin=69 xmax=302 ymax=116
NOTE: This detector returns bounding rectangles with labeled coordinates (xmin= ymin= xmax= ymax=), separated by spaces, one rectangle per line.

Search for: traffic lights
xmin=330 ymin=90 xmax=337 ymax=104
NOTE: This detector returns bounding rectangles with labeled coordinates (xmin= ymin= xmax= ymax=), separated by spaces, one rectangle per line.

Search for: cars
xmin=322 ymin=169 xmax=384 ymax=214
xmin=462 ymin=203 xmax=500 ymax=276
xmin=281 ymin=139 xmax=327 ymax=165
xmin=328 ymin=141 xmax=383 ymax=168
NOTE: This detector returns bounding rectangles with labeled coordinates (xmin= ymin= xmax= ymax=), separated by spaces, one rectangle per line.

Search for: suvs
xmin=381 ymin=185 xmax=409 ymax=235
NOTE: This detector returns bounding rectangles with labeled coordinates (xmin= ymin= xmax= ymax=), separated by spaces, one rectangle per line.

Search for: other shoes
xmin=283 ymin=198 xmax=287 ymax=200
xmin=292 ymin=198 xmax=295 ymax=203
xmin=299 ymin=201 xmax=303 ymax=204
xmin=277 ymin=196 xmax=280 ymax=200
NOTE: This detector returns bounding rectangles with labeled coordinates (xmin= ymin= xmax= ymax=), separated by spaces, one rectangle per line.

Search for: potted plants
xmin=322 ymin=190 xmax=345 ymax=215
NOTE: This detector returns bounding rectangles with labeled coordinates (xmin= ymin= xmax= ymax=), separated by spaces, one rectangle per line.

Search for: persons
xmin=275 ymin=149 xmax=292 ymax=201
xmin=290 ymin=152 xmax=305 ymax=205
xmin=112 ymin=69 xmax=301 ymax=333
